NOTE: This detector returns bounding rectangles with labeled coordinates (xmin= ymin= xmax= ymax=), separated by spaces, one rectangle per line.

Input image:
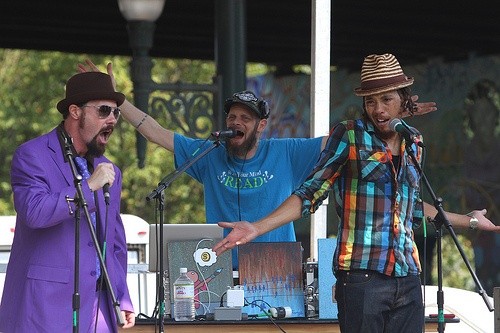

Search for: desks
xmin=114 ymin=315 xmax=460 ymax=332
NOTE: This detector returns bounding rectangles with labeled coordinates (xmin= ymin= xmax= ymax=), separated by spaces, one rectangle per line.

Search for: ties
xmin=74 ymin=157 xmax=101 ymax=279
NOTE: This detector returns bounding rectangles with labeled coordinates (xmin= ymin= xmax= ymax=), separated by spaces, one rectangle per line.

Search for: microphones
xmin=103 ymin=183 xmax=110 ymax=204
xmin=388 ymin=118 xmax=420 ymax=143
xmin=212 ymin=128 xmax=237 ymax=138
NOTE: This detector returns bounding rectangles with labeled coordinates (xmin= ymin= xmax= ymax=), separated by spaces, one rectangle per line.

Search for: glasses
xmin=81 ymin=105 xmax=121 ymax=121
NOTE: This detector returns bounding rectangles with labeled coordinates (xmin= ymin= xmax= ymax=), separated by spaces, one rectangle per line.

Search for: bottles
xmin=172 ymin=267 xmax=196 ymax=322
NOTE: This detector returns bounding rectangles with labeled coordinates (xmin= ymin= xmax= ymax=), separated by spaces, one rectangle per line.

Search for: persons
xmin=78 ymin=57 xmax=437 ymax=270
xmin=211 ymin=53 xmax=500 ymax=333
xmin=0 ymin=71 xmax=136 ymax=333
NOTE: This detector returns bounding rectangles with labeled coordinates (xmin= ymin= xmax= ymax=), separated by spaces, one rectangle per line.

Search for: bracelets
xmin=466 ymin=212 xmax=479 ymax=232
xmin=136 ymin=114 xmax=149 ymax=128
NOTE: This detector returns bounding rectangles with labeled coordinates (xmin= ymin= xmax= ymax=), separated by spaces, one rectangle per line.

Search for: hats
xmin=57 ymin=72 xmax=125 ymax=114
xmin=353 ymin=53 xmax=414 ymax=96
xmin=224 ymin=91 xmax=270 ymax=119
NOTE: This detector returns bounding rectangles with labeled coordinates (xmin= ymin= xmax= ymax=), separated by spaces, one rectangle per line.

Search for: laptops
xmin=148 ymin=224 xmax=224 ymax=272
